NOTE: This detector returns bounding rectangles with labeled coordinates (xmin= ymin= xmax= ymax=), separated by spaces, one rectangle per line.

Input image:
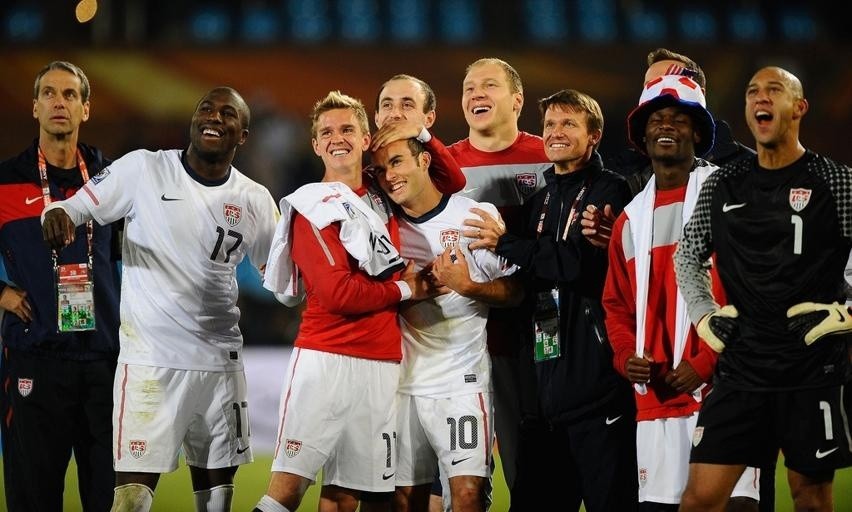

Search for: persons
xmin=602 ymin=72 xmax=761 ymax=512
xmin=463 ymin=89 xmax=634 ymax=511
xmin=1 ymin=61 xmax=127 ymax=512
xmin=40 ymin=86 xmax=308 ymax=512
xmin=671 ymin=66 xmax=852 ymax=512
xmin=371 ymin=137 xmax=521 ymax=512
xmin=581 ymin=49 xmax=780 ymax=512
xmin=443 ymin=59 xmax=554 ymax=511
xmin=361 ymin=75 xmax=495 ymax=512
xmin=253 ymin=90 xmax=451 ymax=512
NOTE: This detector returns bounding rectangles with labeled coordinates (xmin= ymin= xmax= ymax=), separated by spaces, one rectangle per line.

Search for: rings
xmin=477 ymin=231 xmax=480 ymax=238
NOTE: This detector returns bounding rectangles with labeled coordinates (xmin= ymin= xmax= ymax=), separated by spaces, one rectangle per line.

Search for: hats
xmin=626 ymin=75 xmax=716 ymax=159
